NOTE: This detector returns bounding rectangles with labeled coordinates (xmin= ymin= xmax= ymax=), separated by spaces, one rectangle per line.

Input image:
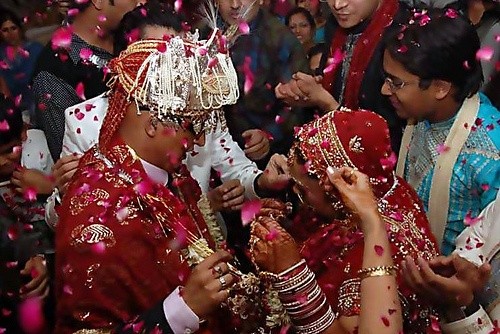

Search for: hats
xmin=294 ymin=107 xmax=396 ymax=213
xmin=109 ymin=36 xmax=240 ymax=118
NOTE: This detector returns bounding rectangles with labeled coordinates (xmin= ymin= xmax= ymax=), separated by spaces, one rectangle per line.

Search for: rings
xmin=227 ymin=288 xmax=235 ymax=296
xmin=213 ymin=266 xmax=222 ymax=277
xmin=220 ymin=277 xmax=227 ymax=289
xmin=249 ymin=237 xmax=260 ymax=247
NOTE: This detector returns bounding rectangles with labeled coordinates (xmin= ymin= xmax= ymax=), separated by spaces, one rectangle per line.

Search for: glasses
xmin=135 ymin=105 xmax=205 ymax=137
xmin=381 ymin=75 xmax=412 ymax=95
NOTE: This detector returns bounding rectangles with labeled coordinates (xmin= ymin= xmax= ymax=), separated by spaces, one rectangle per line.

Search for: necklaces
xmin=158 ymin=186 xmax=215 ymax=255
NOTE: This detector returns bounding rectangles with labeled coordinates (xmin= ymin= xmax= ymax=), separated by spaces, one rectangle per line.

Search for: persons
xmin=249 ymin=106 xmax=439 ymax=334
xmin=247 ymin=0 xmax=500 ymax=256
xmin=1 ymin=1 xmax=291 ymax=334
xmin=399 ymin=190 xmax=500 ymax=334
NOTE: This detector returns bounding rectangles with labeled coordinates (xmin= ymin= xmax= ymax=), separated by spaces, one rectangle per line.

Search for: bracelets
xmin=271 ymin=258 xmax=335 ymax=334
xmin=357 ymin=265 xmax=398 ymax=279
